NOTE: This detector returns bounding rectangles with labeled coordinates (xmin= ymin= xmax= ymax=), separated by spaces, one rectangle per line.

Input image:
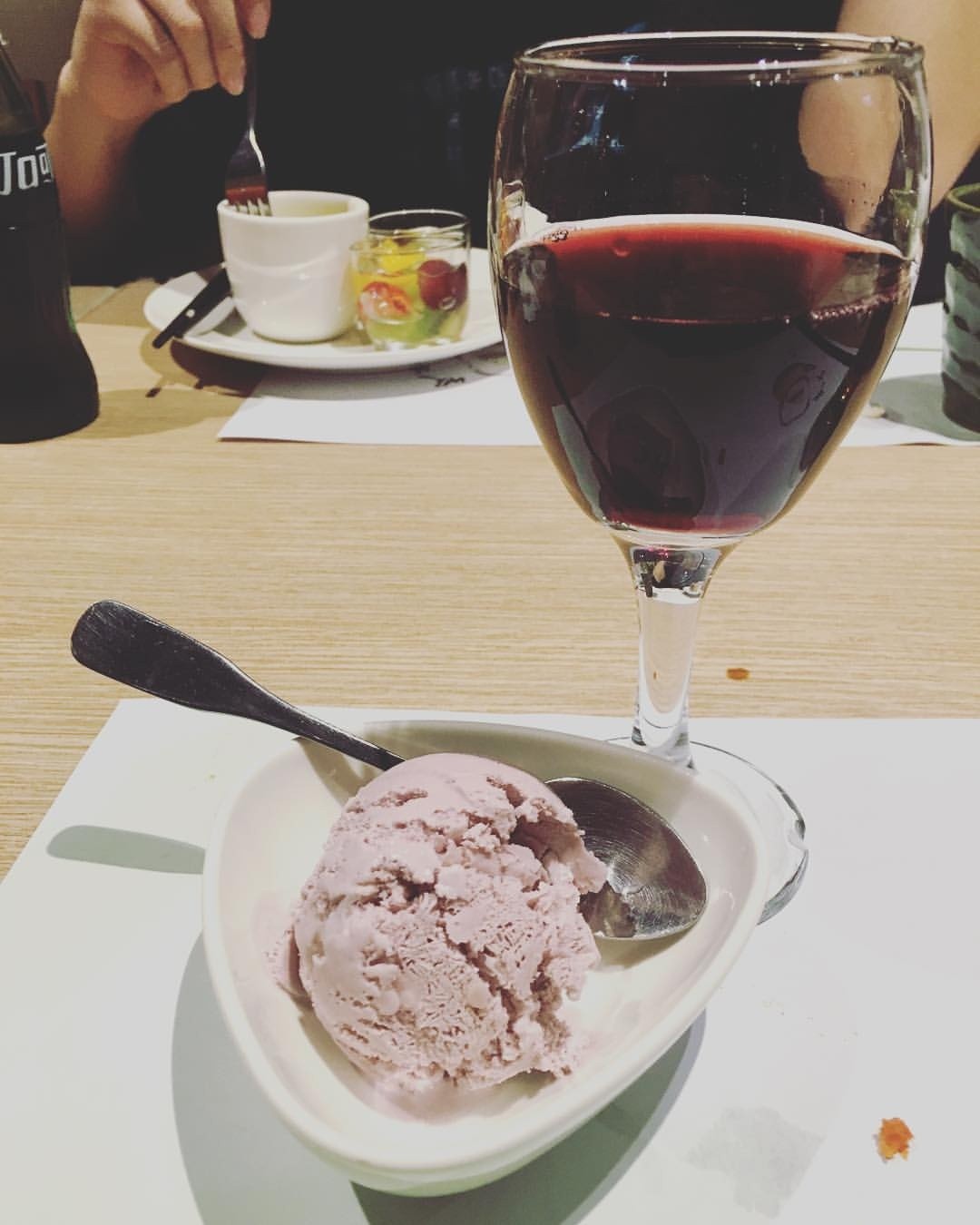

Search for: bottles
xmin=0 ymin=34 xmax=100 ymax=443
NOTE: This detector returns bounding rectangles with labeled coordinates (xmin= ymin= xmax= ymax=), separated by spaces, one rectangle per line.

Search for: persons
xmin=1 ymin=0 xmax=980 ymax=304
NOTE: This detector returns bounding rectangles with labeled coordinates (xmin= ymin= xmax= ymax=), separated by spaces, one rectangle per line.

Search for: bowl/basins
xmin=198 ymin=719 xmax=772 ymax=1199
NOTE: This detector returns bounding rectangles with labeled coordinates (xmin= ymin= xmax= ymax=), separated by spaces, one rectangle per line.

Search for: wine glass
xmin=485 ymin=29 xmax=937 ymax=925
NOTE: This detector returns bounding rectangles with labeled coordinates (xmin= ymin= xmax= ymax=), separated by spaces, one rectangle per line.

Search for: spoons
xmin=69 ymin=597 xmax=709 ymax=943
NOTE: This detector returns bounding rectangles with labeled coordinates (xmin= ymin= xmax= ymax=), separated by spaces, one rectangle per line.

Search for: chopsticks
xmin=151 ymin=268 xmax=231 ymax=349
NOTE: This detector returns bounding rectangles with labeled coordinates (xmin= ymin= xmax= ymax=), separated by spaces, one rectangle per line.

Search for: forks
xmin=223 ymin=33 xmax=273 ymax=217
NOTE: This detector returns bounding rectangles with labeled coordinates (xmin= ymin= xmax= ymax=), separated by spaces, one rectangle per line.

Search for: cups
xmin=216 ymin=189 xmax=370 ymax=344
xmin=348 ymin=235 xmax=470 ymax=353
xmin=367 ymin=207 xmax=471 ymax=251
xmin=940 ymin=182 xmax=980 ymax=434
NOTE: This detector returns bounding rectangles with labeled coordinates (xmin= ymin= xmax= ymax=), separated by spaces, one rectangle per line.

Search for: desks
xmin=0 ymin=286 xmax=980 ymax=1225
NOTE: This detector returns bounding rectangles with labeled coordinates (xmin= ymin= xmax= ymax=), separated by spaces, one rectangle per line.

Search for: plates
xmin=142 ymin=246 xmax=503 ymax=372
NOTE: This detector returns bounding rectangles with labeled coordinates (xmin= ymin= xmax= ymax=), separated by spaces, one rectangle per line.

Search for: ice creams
xmin=292 ymin=752 xmax=608 ymax=1099
